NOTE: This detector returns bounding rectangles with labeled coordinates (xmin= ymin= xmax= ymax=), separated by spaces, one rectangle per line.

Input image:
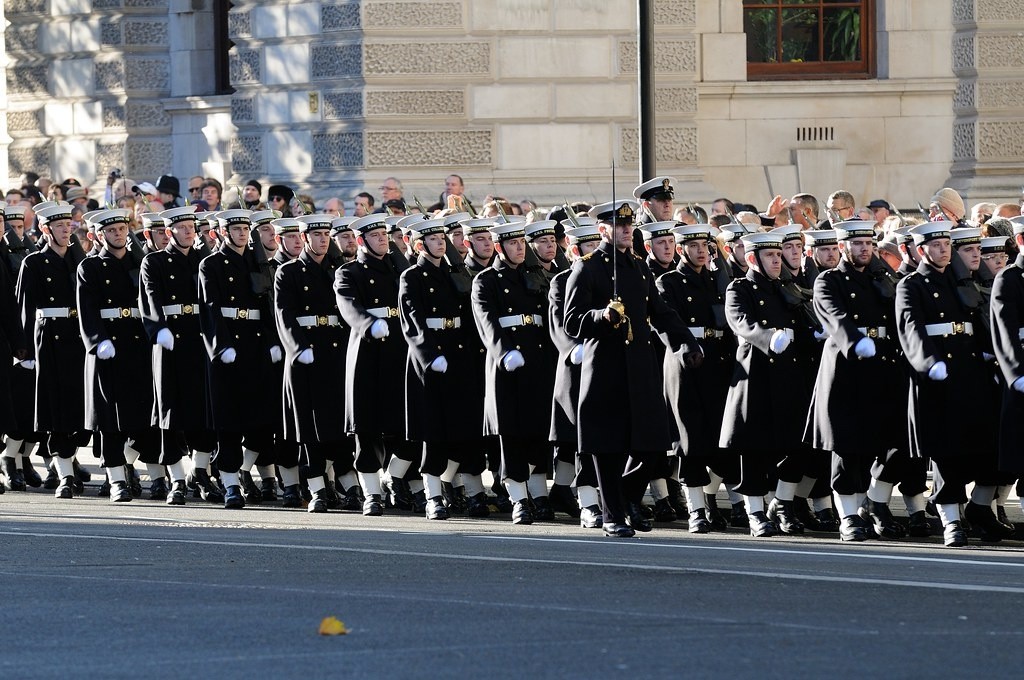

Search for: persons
xmin=0 ymin=172 xmax=1024 ymax=545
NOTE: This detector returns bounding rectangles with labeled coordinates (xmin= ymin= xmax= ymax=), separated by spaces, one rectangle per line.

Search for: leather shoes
xmin=0 ymin=441 xmax=1023 ymax=555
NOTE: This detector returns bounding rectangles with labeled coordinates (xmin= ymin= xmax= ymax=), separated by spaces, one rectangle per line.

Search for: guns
xmin=28 ymin=187 xmax=964 ymax=280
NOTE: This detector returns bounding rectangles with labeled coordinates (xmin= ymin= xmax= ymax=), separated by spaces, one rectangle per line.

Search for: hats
xmin=0 ymin=175 xmax=1021 ymax=276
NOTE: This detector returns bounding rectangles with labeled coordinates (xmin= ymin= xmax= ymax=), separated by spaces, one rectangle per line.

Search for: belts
xmin=33 ymin=301 xmax=1023 ymax=341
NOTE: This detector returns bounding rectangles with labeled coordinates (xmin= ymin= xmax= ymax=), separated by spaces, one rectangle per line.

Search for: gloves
xmin=10 ymin=325 xmax=1023 ymax=405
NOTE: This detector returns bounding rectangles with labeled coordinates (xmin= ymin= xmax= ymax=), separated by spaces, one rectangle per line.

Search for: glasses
xmin=378 ymin=185 xmax=397 ymax=192
xmin=269 ymin=196 xmax=282 ymax=202
xmin=188 ymin=187 xmax=200 ymax=193
xmin=823 ymin=207 xmax=851 ymax=214
xmin=982 ymin=254 xmax=1009 ymax=263
xmin=387 ymin=209 xmax=403 ymax=215
